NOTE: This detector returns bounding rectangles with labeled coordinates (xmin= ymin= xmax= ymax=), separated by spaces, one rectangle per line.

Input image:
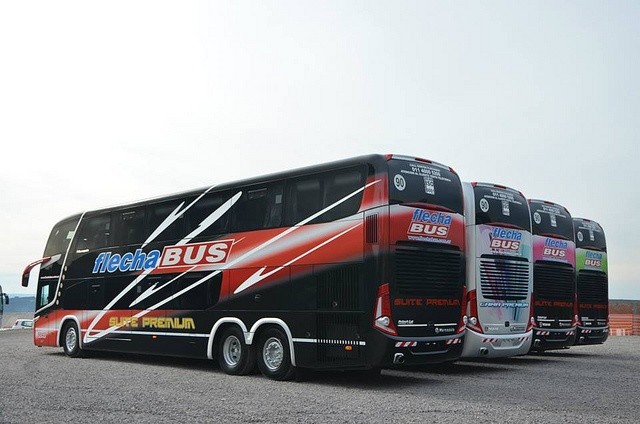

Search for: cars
xmin=13 ymin=319 xmax=33 ymax=328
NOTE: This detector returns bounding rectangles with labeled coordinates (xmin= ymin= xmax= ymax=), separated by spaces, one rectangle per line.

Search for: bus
xmin=461 ymin=181 xmax=533 ymax=358
xmin=22 ymin=154 xmax=466 ymax=380
xmin=572 ymin=217 xmax=609 ymax=346
xmin=528 ymin=198 xmax=578 ymax=353
